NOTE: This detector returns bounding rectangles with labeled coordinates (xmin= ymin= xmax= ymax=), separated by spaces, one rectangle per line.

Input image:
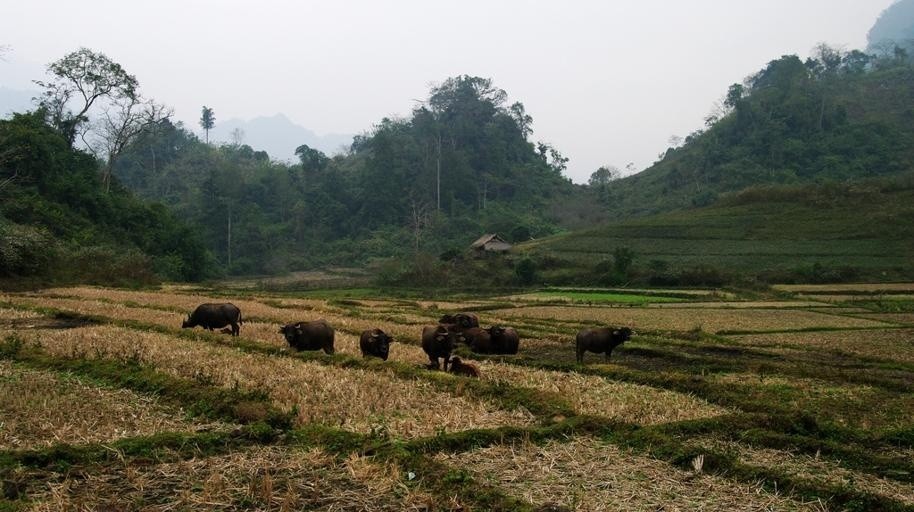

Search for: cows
xmin=576 ymin=326 xmax=632 ymax=364
xmin=361 ymin=329 xmax=394 ymax=361
xmin=181 ymin=303 xmax=242 ymax=337
xmin=421 ymin=312 xmax=519 ymax=377
xmin=278 ymin=319 xmax=334 ymax=355
